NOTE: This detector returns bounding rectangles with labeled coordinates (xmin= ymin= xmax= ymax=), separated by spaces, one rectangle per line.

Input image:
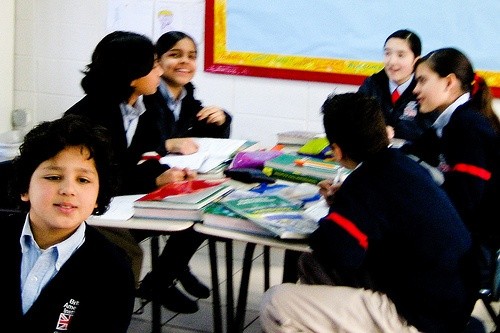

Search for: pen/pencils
xmin=318 ymin=175 xmax=341 ymax=201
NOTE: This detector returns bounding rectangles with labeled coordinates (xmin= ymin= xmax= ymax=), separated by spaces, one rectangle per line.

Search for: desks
xmin=86 ymin=139 xmax=404 ymax=333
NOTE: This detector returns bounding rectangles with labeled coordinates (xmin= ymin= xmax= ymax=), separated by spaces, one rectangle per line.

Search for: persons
xmin=356 ymin=28 xmax=500 ymax=302
xmin=60 ymin=31 xmax=233 ymax=312
xmin=0 ymin=117 xmax=136 ymax=333
xmin=259 ymin=91 xmax=480 ymax=332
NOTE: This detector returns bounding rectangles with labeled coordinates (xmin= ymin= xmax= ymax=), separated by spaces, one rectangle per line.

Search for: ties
xmin=390 ymin=89 xmax=399 ymax=103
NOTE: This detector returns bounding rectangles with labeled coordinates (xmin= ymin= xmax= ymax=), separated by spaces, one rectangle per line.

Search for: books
xmin=133 ymin=131 xmax=349 ymax=240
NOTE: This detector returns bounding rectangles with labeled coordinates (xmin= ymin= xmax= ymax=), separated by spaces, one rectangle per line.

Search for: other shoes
xmin=135 ymin=270 xmax=201 ymax=314
xmin=176 ymin=263 xmax=211 ymax=298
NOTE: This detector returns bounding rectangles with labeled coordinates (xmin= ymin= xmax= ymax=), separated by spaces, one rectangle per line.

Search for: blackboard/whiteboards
xmin=203 ymin=0 xmax=500 ymax=100
